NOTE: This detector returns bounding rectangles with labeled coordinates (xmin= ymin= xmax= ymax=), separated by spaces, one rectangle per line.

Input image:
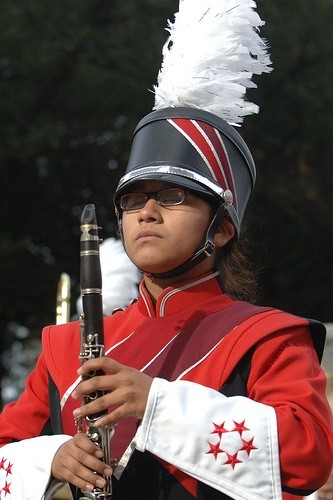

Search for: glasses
xmin=119 ymin=185 xmax=216 ymax=211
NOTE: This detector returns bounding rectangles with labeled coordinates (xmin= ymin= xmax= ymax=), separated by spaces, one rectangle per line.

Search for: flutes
xmin=73 ymin=204 xmax=116 ymax=500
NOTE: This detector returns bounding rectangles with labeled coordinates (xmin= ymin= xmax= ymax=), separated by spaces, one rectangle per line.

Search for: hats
xmin=113 ymin=0 xmax=275 ymax=246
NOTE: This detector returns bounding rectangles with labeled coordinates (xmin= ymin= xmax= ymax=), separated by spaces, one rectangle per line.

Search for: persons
xmin=2 ymin=1 xmax=331 ymax=500
xmin=65 ymin=222 xmax=143 ymax=324
